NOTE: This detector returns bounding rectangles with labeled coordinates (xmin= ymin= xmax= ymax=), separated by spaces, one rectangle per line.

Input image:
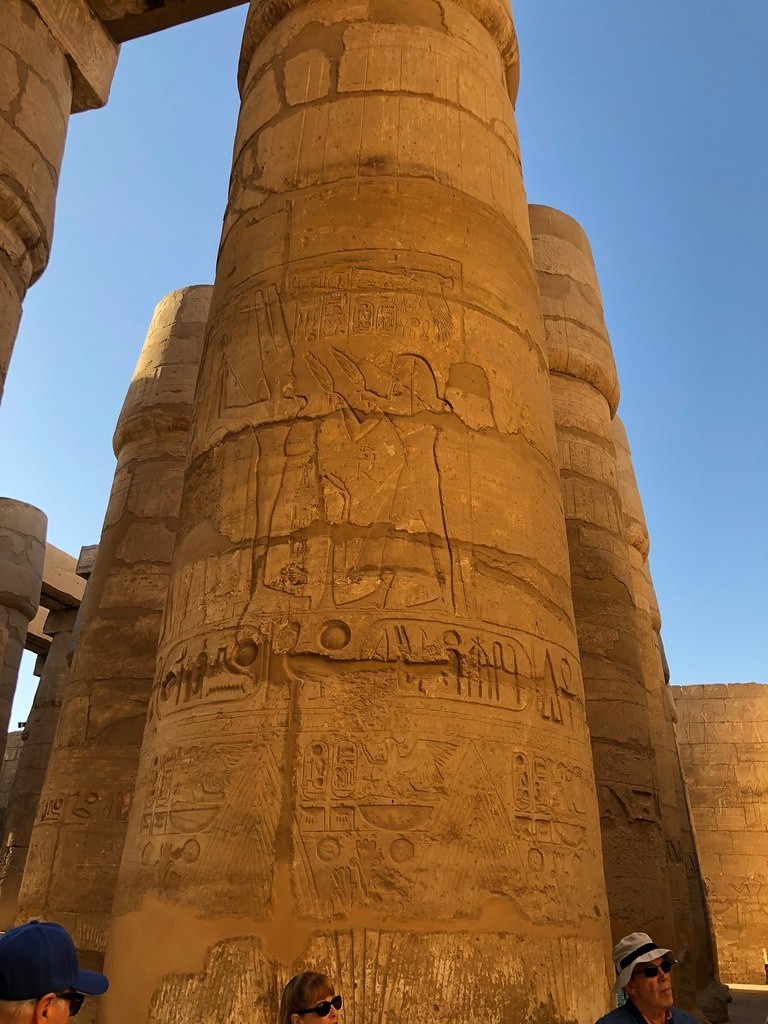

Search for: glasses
xmin=297 ymin=995 xmax=342 ymax=1017
xmin=58 ymin=992 xmax=85 ymax=1016
xmin=632 ymin=960 xmax=671 ymax=978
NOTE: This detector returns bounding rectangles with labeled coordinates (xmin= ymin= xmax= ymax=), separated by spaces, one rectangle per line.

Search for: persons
xmin=594 ymin=933 xmax=700 ymax=1024
xmin=0 ymin=919 xmax=109 ymax=1024
xmin=279 ymin=972 xmax=342 ymax=1024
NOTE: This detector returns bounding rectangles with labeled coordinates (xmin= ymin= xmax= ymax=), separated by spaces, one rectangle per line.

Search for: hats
xmin=612 ymin=932 xmax=677 ymax=993
xmin=0 ymin=920 xmax=109 ymax=1001
